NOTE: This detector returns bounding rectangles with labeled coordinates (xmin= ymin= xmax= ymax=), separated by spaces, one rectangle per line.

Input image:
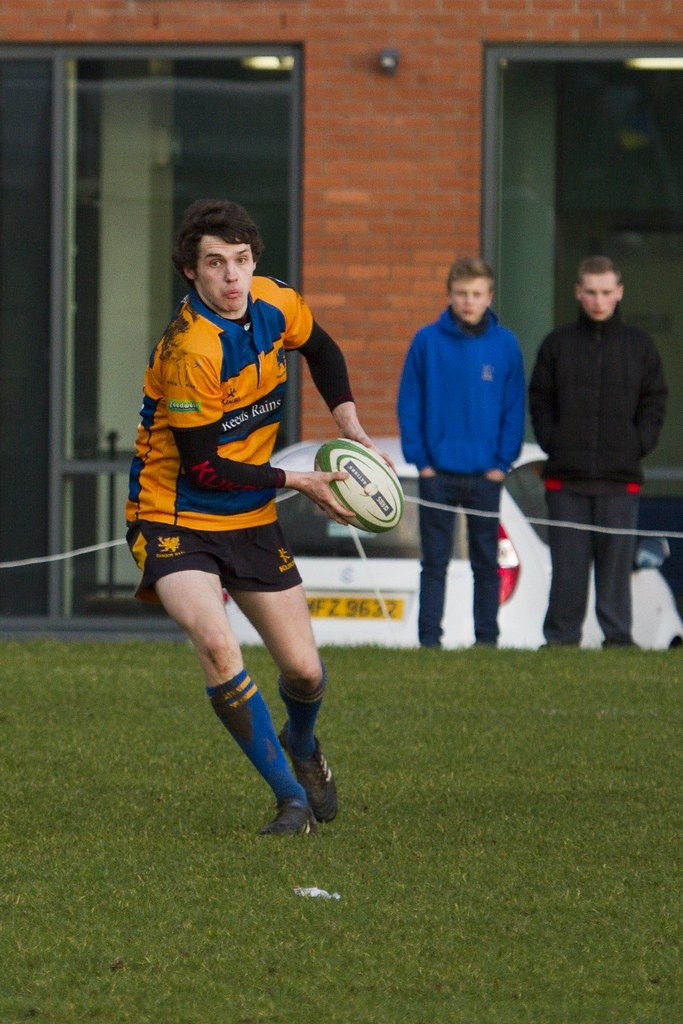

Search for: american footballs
xmin=314 ymin=436 xmax=405 ymax=535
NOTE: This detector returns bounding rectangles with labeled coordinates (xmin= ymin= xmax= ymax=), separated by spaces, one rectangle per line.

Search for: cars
xmin=218 ymin=434 xmax=683 ymax=651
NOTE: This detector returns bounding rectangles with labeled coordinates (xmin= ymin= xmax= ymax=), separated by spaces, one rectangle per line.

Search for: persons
xmin=397 ymin=257 xmax=527 ymax=648
xmin=126 ymin=201 xmax=396 ymax=836
xmin=528 ymin=255 xmax=669 ymax=650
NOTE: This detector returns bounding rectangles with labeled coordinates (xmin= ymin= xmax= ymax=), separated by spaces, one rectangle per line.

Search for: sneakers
xmin=278 ymin=720 xmax=338 ymax=823
xmin=259 ymin=800 xmax=317 ymax=837
